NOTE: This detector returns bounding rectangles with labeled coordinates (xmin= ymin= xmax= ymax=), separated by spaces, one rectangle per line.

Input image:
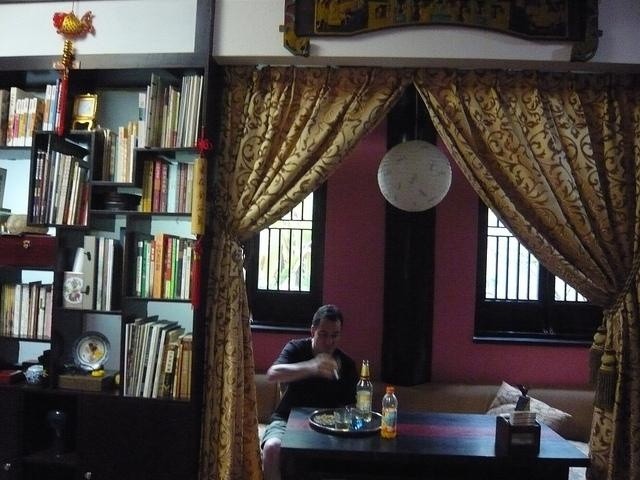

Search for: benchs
xmin=254 ymin=376 xmax=595 ymax=480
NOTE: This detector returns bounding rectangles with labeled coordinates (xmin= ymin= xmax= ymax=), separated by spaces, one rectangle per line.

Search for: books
xmin=123 ymin=315 xmax=193 ymax=401
xmin=93 ymin=120 xmax=138 ymax=184
xmin=1 ymin=78 xmax=63 ymax=147
xmin=141 ymin=155 xmax=202 ymax=235
xmin=1 ymin=280 xmax=54 ymax=340
xmin=129 ymin=232 xmax=193 ymax=300
xmin=82 ymin=234 xmax=120 ymax=312
xmin=31 ymin=149 xmax=90 ymax=226
xmin=138 ymin=73 xmax=203 ymax=149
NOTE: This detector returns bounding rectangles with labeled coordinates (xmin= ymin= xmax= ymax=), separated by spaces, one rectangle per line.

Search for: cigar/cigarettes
xmin=334 ymin=368 xmax=340 ymax=381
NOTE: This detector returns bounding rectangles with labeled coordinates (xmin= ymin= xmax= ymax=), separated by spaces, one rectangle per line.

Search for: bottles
xmin=381 ymin=386 xmax=398 ymax=440
xmin=355 ymin=360 xmax=374 ymax=423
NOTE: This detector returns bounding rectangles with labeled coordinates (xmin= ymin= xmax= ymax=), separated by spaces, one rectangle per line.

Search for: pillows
xmin=486 ymin=380 xmax=573 ymax=432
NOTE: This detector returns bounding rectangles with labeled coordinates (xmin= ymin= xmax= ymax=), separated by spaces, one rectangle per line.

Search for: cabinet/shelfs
xmin=0 ymin=53 xmax=220 ymax=480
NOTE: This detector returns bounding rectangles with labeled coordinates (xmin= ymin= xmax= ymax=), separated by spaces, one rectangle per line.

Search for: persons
xmin=260 ymin=304 xmax=360 ymax=480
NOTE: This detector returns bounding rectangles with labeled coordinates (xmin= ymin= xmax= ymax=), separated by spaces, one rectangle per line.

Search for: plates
xmin=72 ymin=331 xmax=111 ymax=372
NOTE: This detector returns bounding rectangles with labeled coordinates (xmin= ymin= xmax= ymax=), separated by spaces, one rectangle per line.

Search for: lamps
xmin=377 ymin=89 xmax=452 ymax=213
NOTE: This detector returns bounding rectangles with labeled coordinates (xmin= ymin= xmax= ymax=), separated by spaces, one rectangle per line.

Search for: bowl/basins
xmin=99 ymin=191 xmax=142 ymax=209
xmin=5 ymin=214 xmax=50 ymax=237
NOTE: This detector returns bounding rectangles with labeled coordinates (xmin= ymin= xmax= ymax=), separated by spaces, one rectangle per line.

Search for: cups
xmin=25 ymin=365 xmax=44 ymax=385
xmin=345 ymin=403 xmax=356 ymax=419
xmin=333 ymin=409 xmax=352 ymax=431
